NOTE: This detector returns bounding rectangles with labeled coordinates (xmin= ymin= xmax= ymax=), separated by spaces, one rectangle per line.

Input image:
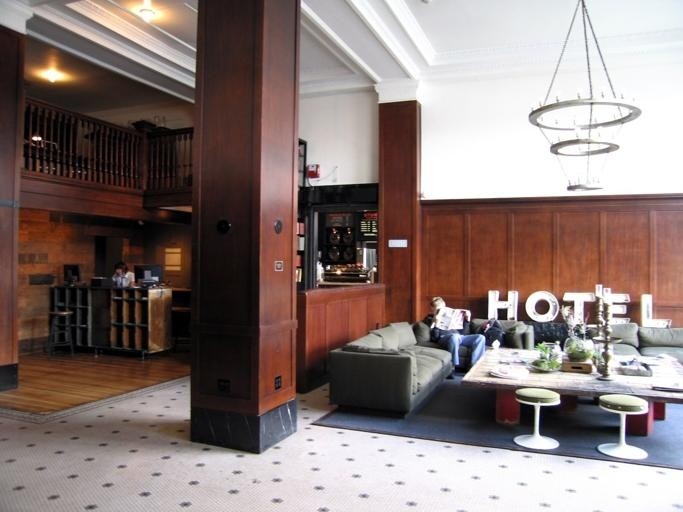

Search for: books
xmin=611 ymin=352 xmax=683 ymax=400
xmin=484 ymin=347 xmax=536 ymax=380
xmin=433 ymin=306 xmax=469 ymax=333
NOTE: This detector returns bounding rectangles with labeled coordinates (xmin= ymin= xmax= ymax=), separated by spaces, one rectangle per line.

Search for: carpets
xmin=311 ymin=373 xmax=682 ymax=470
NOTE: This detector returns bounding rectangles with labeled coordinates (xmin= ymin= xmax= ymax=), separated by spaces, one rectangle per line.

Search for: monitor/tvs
xmin=134 ymin=263 xmax=163 ymax=283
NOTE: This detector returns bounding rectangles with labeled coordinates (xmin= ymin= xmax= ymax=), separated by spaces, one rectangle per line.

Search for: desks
xmin=462 ymin=348 xmax=681 ymax=437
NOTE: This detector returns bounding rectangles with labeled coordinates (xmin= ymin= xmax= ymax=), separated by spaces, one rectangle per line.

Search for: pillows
xmin=479 ymin=317 xmax=504 ymax=347
xmin=505 ymin=320 xmax=527 ymax=351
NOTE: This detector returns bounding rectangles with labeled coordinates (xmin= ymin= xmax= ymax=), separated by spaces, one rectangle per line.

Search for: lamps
xmin=527 ymin=0 xmax=642 ymax=192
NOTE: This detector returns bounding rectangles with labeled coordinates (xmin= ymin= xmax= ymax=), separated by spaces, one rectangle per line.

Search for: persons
xmin=428 ymin=295 xmax=487 ymax=372
xmin=111 ymin=261 xmax=136 ymax=290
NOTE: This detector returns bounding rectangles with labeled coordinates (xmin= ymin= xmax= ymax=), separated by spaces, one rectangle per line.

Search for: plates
xmin=489 ymin=363 xmax=528 ymax=381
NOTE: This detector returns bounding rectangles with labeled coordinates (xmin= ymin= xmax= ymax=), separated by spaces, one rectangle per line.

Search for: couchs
xmin=330 ymin=317 xmax=681 ymax=414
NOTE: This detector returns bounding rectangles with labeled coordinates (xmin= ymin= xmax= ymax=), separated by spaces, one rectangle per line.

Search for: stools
xmin=596 ymin=390 xmax=650 ymax=461
xmin=512 ymin=386 xmax=562 ymax=452
xmin=43 ymin=310 xmax=77 ymax=360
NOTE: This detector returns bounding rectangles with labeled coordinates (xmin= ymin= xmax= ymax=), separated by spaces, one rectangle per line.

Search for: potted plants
xmin=558 ymin=302 xmax=595 ymax=363
